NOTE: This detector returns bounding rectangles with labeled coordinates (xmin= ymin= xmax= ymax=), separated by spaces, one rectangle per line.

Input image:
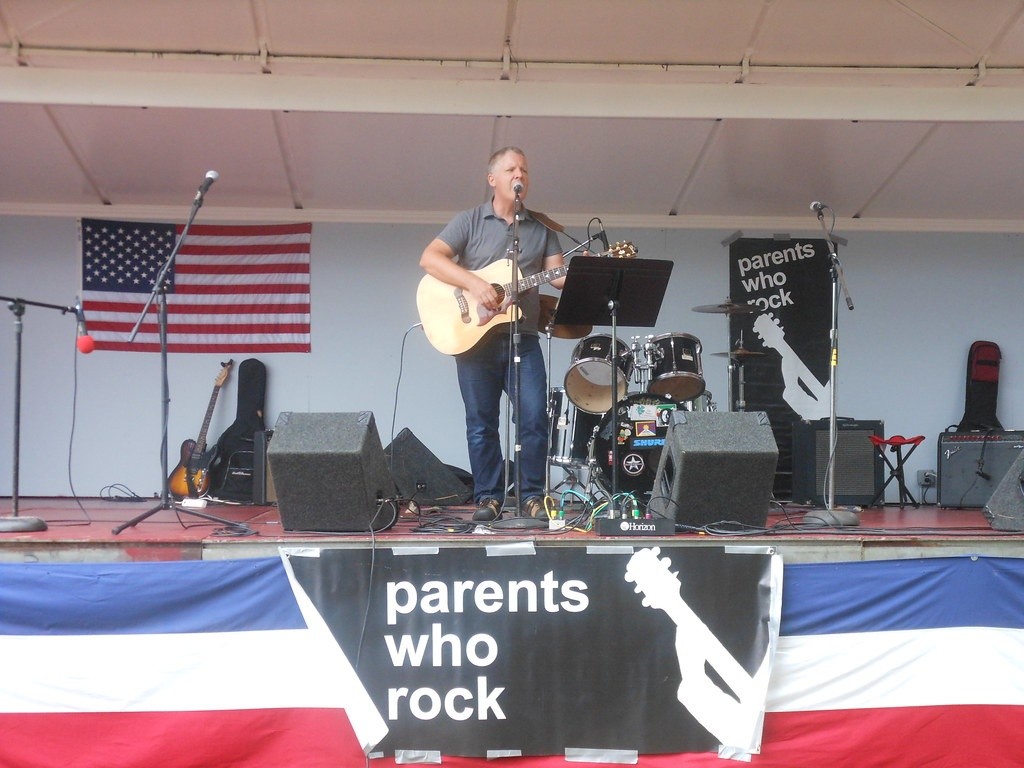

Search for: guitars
xmin=167 ymin=358 xmax=234 ymax=500
xmin=416 ymin=239 xmax=640 ymax=358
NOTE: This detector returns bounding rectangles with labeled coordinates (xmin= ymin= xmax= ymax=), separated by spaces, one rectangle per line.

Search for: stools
xmin=868 ymin=435 xmax=925 ymax=509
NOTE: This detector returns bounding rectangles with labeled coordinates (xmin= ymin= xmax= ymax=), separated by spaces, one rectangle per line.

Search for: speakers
xmin=382 ymin=427 xmax=474 ymax=508
xmin=937 ymin=431 xmax=1024 ymax=532
xmin=648 ymin=410 xmax=779 ymax=533
xmin=252 ymin=430 xmax=278 ymax=506
xmin=266 ymin=412 xmax=399 ymax=531
xmin=791 ymin=420 xmax=886 ymax=507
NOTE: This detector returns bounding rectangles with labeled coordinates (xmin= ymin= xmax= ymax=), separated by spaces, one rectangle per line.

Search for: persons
xmin=420 ymin=146 xmax=589 ymax=519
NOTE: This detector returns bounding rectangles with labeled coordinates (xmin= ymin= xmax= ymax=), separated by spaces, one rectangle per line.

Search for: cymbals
xmin=536 ymin=293 xmax=593 ymax=339
xmin=691 ymin=303 xmax=766 ymax=315
xmin=709 ymin=349 xmax=769 ymax=360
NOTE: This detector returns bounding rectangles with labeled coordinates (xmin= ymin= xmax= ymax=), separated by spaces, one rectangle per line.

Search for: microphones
xmin=599 ymin=218 xmax=610 ymax=252
xmin=76 ymin=299 xmax=95 ymax=353
xmin=513 ymin=182 xmax=525 ymax=194
xmin=196 ymin=170 xmax=219 ymax=201
xmin=810 ymin=201 xmax=826 ymax=211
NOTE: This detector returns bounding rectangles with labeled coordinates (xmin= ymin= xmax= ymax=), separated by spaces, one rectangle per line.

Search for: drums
xmin=544 ymin=387 xmax=604 ymax=471
xmin=680 ymin=390 xmax=713 ymax=412
xmin=642 ymin=332 xmax=707 ymax=402
xmin=563 ymin=334 xmax=637 ymax=415
xmin=588 ymin=392 xmax=690 ymax=508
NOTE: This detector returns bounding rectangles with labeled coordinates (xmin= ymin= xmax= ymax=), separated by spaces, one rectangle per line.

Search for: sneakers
xmin=514 ymin=497 xmax=551 ymax=521
xmin=472 ymin=497 xmax=503 ymax=520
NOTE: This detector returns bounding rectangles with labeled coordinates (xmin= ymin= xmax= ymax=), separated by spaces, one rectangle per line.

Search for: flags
xmin=80 ymin=217 xmax=312 ymax=354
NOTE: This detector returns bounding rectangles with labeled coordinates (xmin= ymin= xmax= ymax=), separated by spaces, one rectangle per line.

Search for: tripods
xmin=112 ymin=194 xmax=249 ymax=536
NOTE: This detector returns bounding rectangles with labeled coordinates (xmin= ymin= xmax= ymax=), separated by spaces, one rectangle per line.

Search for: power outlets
xmin=917 ymin=470 xmax=935 ymax=486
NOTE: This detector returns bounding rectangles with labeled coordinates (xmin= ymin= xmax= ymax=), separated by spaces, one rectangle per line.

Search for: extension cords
xmin=182 ymin=497 xmax=208 ymax=509
